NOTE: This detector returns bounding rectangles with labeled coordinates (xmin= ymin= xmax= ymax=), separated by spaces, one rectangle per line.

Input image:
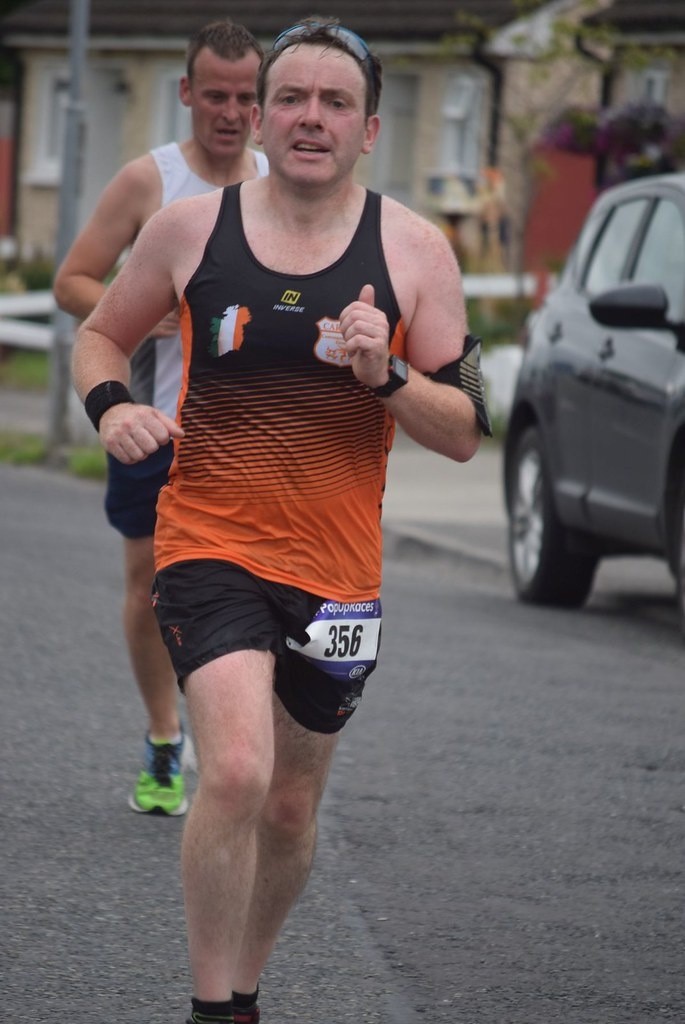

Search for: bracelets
xmin=83 ymin=379 xmax=135 ymax=434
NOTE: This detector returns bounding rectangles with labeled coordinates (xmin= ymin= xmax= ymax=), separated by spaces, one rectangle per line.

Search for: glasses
xmin=272 ymin=22 xmax=370 ymax=62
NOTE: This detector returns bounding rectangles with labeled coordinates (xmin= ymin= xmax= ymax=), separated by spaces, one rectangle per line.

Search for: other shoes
xmin=185 ymin=1005 xmax=261 ymax=1024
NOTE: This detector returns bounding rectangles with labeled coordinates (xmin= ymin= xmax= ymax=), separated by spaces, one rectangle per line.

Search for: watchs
xmin=370 ymin=355 xmax=409 ymax=398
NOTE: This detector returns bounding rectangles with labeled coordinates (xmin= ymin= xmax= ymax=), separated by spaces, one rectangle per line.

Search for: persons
xmin=52 ymin=20 xmax=270 ymax=818
xmin=69 ymin=14 xmax=484 ymax=1024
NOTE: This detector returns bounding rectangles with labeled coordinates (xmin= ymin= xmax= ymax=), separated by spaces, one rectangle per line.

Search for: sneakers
xmin=126 ymin=726 xmax=195 ymax=817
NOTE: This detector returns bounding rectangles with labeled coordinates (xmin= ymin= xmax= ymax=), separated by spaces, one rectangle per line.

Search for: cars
xmin=502 ymin=175 xmax=685 ymax=612
